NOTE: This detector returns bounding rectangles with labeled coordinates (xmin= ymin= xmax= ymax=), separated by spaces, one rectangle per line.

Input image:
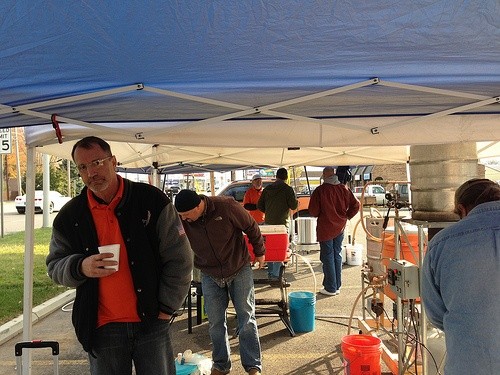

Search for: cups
xmin=99 ymin=244 xmax=120 ymax=271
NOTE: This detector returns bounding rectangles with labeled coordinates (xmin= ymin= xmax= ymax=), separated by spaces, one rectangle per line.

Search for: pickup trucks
xmin=353 ymin=185 xmax=393 ymax=205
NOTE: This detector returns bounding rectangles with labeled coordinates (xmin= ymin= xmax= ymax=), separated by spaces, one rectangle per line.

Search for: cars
xmin=217 ymin=180 xmax=308 ymax=232
xmin=398 ymin=185 xmax=408 ymax=202
xmin=15 ymin=190 xmax=72 ymax=214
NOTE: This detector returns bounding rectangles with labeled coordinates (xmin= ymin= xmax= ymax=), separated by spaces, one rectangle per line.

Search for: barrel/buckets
xmin=296 ymin=217 xmax=317 ymax=244
xmin=288 ymin=293 xmax=316 ymax=332
xmin=347 ymin=244 xmax=363 ymax=266
xmin=383 ymin=231 xmax=428 ymax=303
xmin=411 ymin=143 xmax=477 ymax=222
xmin=341 ymin=334 xmax=381 ymax=375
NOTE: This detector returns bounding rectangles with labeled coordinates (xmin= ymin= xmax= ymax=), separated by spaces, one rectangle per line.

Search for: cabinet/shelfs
xmin=187 ymin=259 xmax=297 ymax=333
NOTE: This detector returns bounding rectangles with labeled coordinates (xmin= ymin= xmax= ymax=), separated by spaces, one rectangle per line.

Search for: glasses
xmin=76 ymin=156 xmax=112 ymax=171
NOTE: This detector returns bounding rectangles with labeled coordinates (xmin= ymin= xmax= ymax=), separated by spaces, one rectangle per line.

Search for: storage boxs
xmin=242 ymin=225 xmax=288 ymax=261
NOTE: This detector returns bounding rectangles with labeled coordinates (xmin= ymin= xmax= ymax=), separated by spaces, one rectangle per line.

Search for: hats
xmin=174 ymin=189 xmax=201 ymax=213
xmin=453 ymin=178 xmax=495 ymax=213
xmin=252 ymin=174 xmax=261 ymax=180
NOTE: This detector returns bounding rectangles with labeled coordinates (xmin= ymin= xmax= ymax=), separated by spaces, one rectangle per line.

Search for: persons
xmin=173 ymin=189 xmax=266 ymax=375
xmin=243 ymin=168 xmax=299 ymax=287
xmin=419 ymin=178 xmax=500 ymax=375
xmin=308 ymin=166 xmax=360 ymax=296
xmin=45 ymin=136 xmax=194 ymax=375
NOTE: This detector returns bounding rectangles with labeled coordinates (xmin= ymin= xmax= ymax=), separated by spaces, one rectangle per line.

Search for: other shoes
xmin=269 ymin=281 xmax=290 ymax=287
xmin=319 ymin=286 xmax=340 ymax=295
xmin=210 ymin=369 xmax=230 ymax=375
xmin=249 ymin=369 xmax=261 ymax=375
